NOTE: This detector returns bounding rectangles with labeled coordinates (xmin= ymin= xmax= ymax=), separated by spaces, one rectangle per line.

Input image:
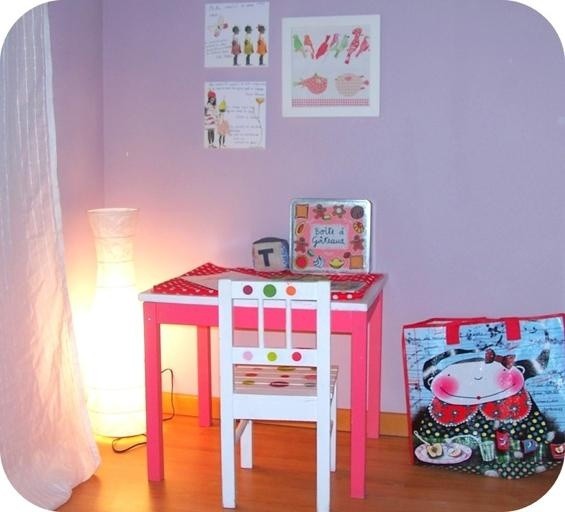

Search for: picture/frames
xmin=281 ymin=12 xmax=382 ymax=121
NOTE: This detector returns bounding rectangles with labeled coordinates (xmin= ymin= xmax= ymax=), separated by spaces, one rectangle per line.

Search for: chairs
xmin=217 ymin=276 xmax=341 ymax=512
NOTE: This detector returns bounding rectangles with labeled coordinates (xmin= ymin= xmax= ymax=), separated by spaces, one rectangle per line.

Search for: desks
xmin=139 ymin=267 xmax=385 ymax=501
